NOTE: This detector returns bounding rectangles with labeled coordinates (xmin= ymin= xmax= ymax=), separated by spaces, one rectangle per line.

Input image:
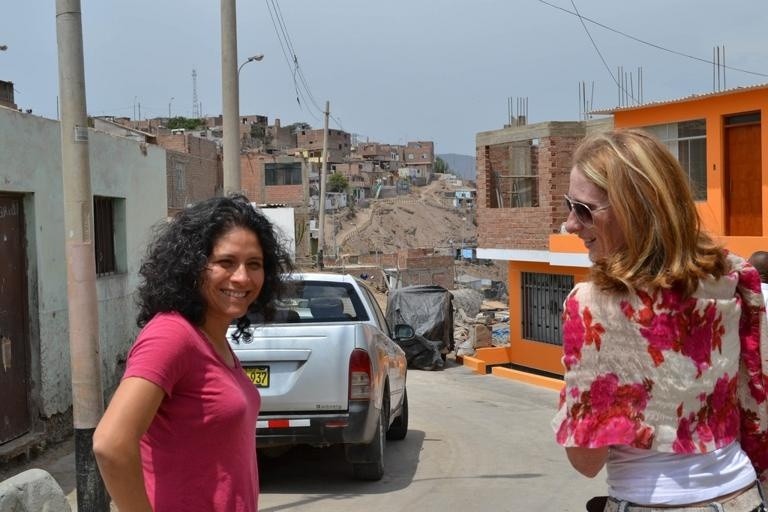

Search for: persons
xmin=547 ymin=125 xmax=767 ymax=511
xmin=89 ymin=190 xmax=302 ymax=511
xmin=745 ymin=249 xmax=768 ymax=310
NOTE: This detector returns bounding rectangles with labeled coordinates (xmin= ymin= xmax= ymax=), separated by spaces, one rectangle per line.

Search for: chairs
xmin=309 ymin=299 xmax=353 ymax=319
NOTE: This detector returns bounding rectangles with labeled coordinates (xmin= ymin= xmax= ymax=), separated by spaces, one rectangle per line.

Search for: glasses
xmin=562 ymin=192 xmax=611 ymax=231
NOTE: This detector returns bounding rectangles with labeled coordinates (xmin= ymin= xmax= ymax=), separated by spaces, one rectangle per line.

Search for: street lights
xmin=238 ymin=55 xmax=263 ymax=75
xmin=133 ymin=96 xmax=137 ymax=128
xmin=169 ymin=97 xmax=175 ymax=118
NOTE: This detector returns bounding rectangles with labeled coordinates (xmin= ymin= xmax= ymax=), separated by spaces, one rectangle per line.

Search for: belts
xmin=586 ymin=483 xmax=765 ymax=512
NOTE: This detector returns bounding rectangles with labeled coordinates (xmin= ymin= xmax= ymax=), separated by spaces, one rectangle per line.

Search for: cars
xmin=225 ymin=273 xmax=415 ymax=481
